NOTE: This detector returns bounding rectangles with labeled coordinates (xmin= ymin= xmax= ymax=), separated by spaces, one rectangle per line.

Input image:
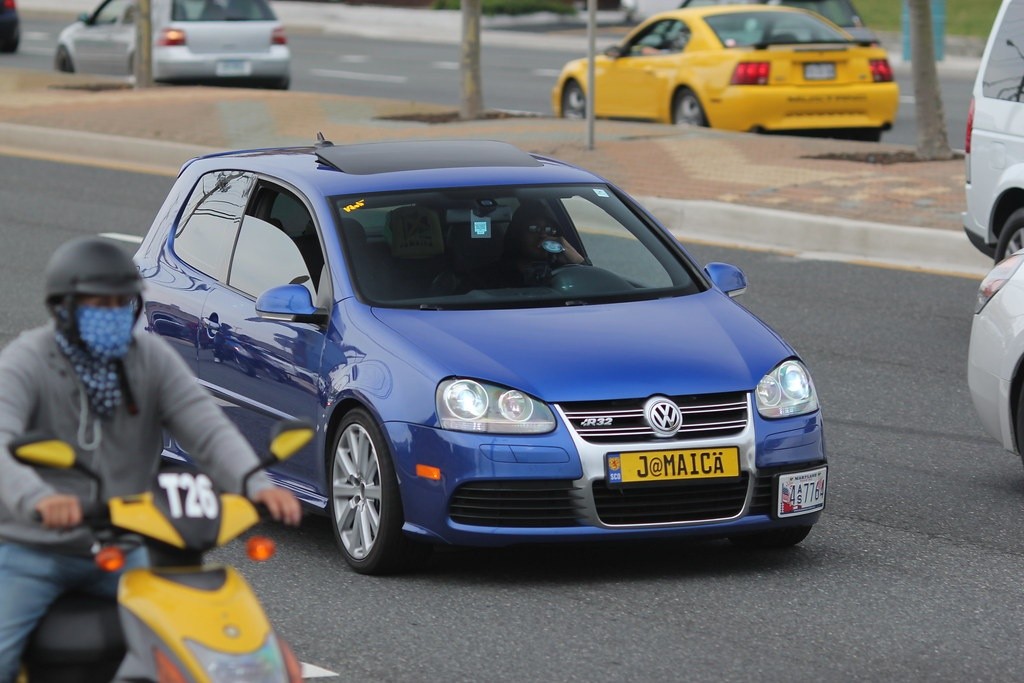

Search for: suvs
xmin=960 ymin=0 xmax=1023 ymax=267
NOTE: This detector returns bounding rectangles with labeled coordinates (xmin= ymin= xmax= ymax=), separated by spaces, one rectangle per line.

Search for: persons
xmin=0 ymin=236 xmax=304 ymax=683
xmin=494 ymin=202 xmax=593 ymax=287
xmin=640 ymin=28 xmax=688 ymax=56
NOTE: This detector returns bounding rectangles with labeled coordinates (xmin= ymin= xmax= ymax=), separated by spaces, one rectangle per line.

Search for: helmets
xmin=40 ymin=236 xmax=146 ymax=299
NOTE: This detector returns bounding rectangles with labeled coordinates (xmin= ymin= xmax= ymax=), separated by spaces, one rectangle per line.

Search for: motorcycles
xmin=1 ymin=420 xmax=318 ymax=683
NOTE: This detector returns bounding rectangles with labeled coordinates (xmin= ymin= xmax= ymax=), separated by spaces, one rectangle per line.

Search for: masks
xmin=60 ymin=304 xmax=133 ymax=360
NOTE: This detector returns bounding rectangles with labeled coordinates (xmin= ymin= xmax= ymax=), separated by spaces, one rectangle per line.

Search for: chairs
xmin=265 ymin=204 xmax=511 ymax=300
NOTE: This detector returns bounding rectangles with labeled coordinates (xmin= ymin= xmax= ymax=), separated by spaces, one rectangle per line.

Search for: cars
xmin=129 ymin=141 xmax=831 ymax=575
xmin=552 ymin=3 xmax=901 ymax=144
xmin=54 ymin=0 xmax=293 ymax=91
xmin=660 ymin=0 xmax=884 ymax=53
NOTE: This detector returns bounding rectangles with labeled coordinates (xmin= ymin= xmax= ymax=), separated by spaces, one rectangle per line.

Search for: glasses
xmin=524 ymin=223 xmax=557 ymax=236
